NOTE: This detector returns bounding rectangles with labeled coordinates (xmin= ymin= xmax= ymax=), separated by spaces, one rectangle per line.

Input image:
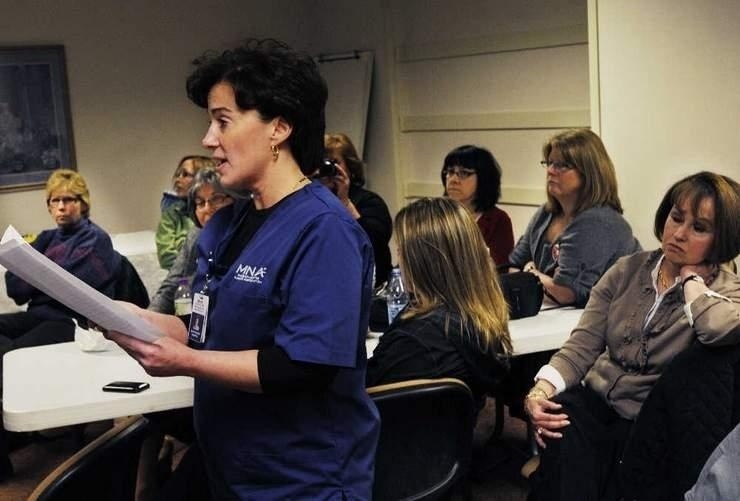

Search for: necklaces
xmin=287 ymin=175 xmax=307 ymax=198
xmin=655 ymin=268 xmax=671 ymax=291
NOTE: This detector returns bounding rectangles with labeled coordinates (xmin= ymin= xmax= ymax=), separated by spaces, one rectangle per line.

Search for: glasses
xmin=540 ymin=161 xmax=577 ymax=172
xmin=194 ymin=193 xmax=231 ymax=208
xmin=172 ymin=168 xmax=195 ymax=178
xmin=443 ymin=169 xmax=476 ymax=179
xmin=50 ymin=196 xmax=81 ymax=204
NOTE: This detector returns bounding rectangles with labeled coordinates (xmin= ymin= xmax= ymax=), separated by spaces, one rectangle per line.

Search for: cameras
xmin=311 ymin=158 xmax=340 ymax=179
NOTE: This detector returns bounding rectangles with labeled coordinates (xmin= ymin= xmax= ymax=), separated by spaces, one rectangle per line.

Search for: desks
xmin=2 ymin=305 xmax=585 ymax=501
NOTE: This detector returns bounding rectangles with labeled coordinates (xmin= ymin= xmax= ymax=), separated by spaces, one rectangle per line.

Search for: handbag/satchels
xmin=499 ymin=271 xmax=560 ymax=320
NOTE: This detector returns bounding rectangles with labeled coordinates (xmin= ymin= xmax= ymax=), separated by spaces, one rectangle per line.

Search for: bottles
xmin=174 ymin=278 xmax=191 ymax=331
xmin=388 ymin=269 xmax=410 ymax=325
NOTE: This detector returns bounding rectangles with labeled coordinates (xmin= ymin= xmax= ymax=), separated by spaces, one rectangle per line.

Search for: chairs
xmin=365 ymin=378 xmax=476 ymax=500
xmin=27 ymin=414 xmax=150 ymax=501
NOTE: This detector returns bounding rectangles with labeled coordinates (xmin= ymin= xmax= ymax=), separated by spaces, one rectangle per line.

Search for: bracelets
xmin=345 ymin=199 xmax=350 ymax=207
xmin=680 ymin=274 xmax=703 ymax=289
xmin=523 ymin=386 xmax=545 ymax=415
xmin=523 ymin=266 xmax=536 ymax=272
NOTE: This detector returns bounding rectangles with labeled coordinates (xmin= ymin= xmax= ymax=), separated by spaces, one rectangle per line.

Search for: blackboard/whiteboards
xmin=311 ymin=50 xmax=374 ymax=162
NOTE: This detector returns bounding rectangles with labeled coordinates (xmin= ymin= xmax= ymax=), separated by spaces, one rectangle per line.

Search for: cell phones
xmin=103 ymin=381 xmax=149 ymax=393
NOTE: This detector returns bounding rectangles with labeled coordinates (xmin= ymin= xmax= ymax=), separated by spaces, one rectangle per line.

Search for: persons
xmin=364 ymin=198 xmax=514 ymax=501
xmin=480 ymin=126 xmax=641 ymax=420
xmin=312 ymin=134 xmax=393 ymax=288
xmin=157 ymin=153 xmax=214 ymax=269
xmin=89 ymin=35 xmax=381 ymax=501
xmin=0 ymin=169 xmax=115 ymax=485
xmin=524 ymin=172 xmax=740 ymax=501
xmin=441 ymin=145 xmax=514 ymax=272
xmin=146 ymin=165 xmax=247 ymax=312
